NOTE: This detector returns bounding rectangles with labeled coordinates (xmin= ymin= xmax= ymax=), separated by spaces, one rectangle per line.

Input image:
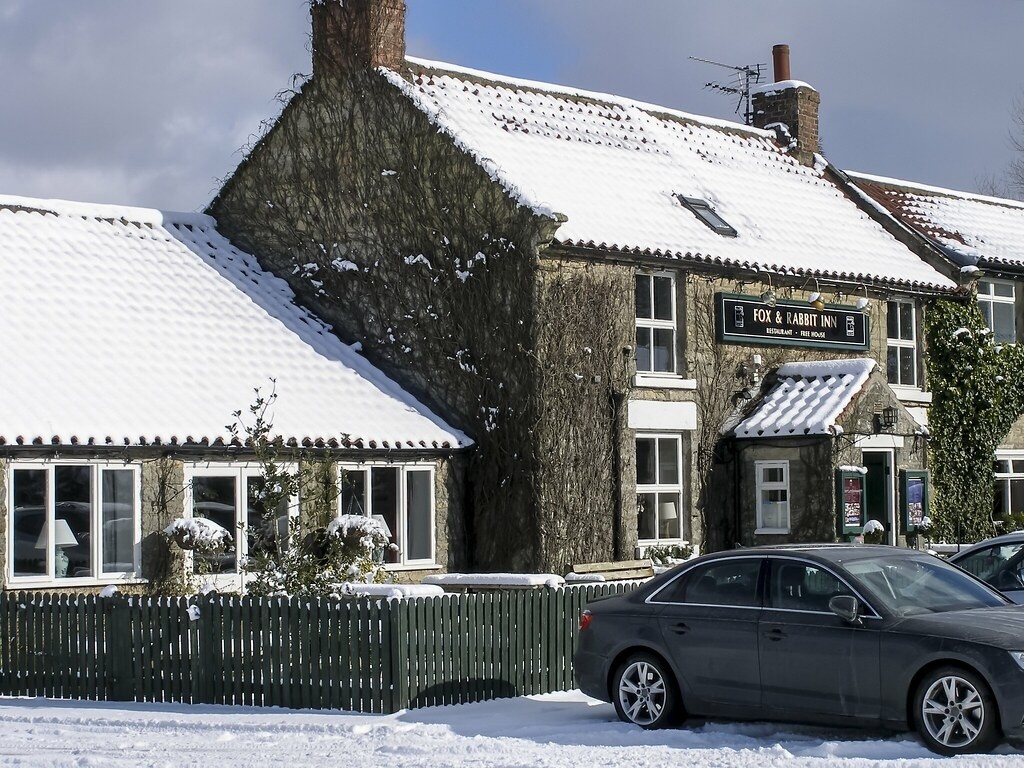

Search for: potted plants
xmin=323 ymin=515 xmax=403 ymax=557
xmin=163 ymin=516 xmax=234 ymax=549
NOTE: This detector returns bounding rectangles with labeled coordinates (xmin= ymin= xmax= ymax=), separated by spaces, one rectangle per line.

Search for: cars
xmin=573 ymin=543 xmax=1024 ymax=757
xmin=900 ymin=530 xmax=1024 ymax=671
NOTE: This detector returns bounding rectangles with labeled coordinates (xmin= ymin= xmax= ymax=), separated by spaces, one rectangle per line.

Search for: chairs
xmin=781 ymin=564 xmax=820 ymax=614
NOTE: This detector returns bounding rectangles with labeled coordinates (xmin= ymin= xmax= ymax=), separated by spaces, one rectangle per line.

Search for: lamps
xmin=742 ymin=273 xmax=777 ymax=307
xmin=658 ymin=502 xmax=678 ymax=538
xmin=841 ymin=283 xmax=872 ymax=315
xmin=34 ymin=519 xmax=79 ymax=579
xmin=790 ymin=278 xmax=824 ymax=311
xmin=872 ymin=405 xmax=899 ymax=434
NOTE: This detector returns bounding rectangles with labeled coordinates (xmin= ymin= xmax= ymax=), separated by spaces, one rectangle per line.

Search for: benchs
xmin=695 ymin=576 xmax=756 ymax=605
